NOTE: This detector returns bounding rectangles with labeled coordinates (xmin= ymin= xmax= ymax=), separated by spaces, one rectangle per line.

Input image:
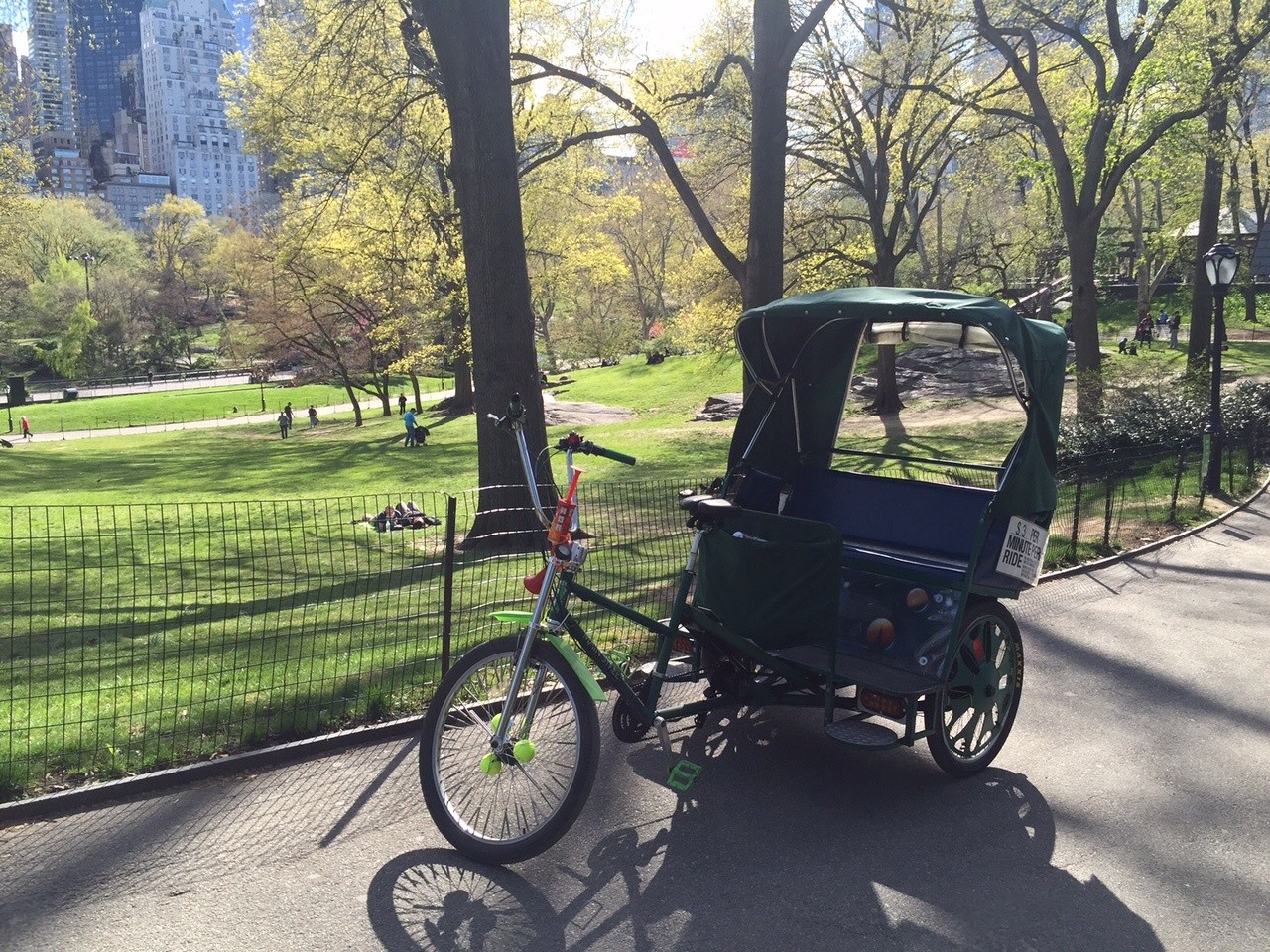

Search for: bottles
xmin=386 ymin=520 xmax=390 ymax=533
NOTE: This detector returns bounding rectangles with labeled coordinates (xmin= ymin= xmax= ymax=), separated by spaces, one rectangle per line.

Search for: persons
xmin=283 ymin=401 xmax=295 ymax=429
xmin=404 ymin=408 xmax=418 ymax=448
xmin=1052 ymin=319 xmax=1074 ymax=345
xmin=147 ymin=370 xmax=154 ymax=386
xmin=20 ymin=416 xmax=33 ymax=439
xmin=644 ymin=349 xmax=664 ymax=366
xmin=601 ymin=357 xmax=621 ymax=367
xmin=399 ymin=392 xmax=408 ymax=416
xmin=276 ymin=411 xmax=288 ymax=439
xmin=1119 ymin=309 xmax=1180 ymax=356
xmin=307 ymin=404 xmax=319 ymax=429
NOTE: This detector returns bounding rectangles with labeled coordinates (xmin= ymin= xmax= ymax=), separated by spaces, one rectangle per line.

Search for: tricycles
xmin=417 ymin=282 xmax=1068 ymax=862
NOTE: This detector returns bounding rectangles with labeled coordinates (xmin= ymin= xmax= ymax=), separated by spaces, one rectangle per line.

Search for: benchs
xmin=734 ymin=459 xmax=1008 ymax=593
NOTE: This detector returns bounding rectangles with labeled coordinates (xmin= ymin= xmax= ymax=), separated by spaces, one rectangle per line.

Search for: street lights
xmin=257 ymin=368 xmax=266 ymax=410
xmin=1200 ymin=239 xmax=1242 ymax=489
xmin=67 ymin=253 xmax=95 ymax=298
xmin=3 ymin=384 xmax=13 ymax=432
